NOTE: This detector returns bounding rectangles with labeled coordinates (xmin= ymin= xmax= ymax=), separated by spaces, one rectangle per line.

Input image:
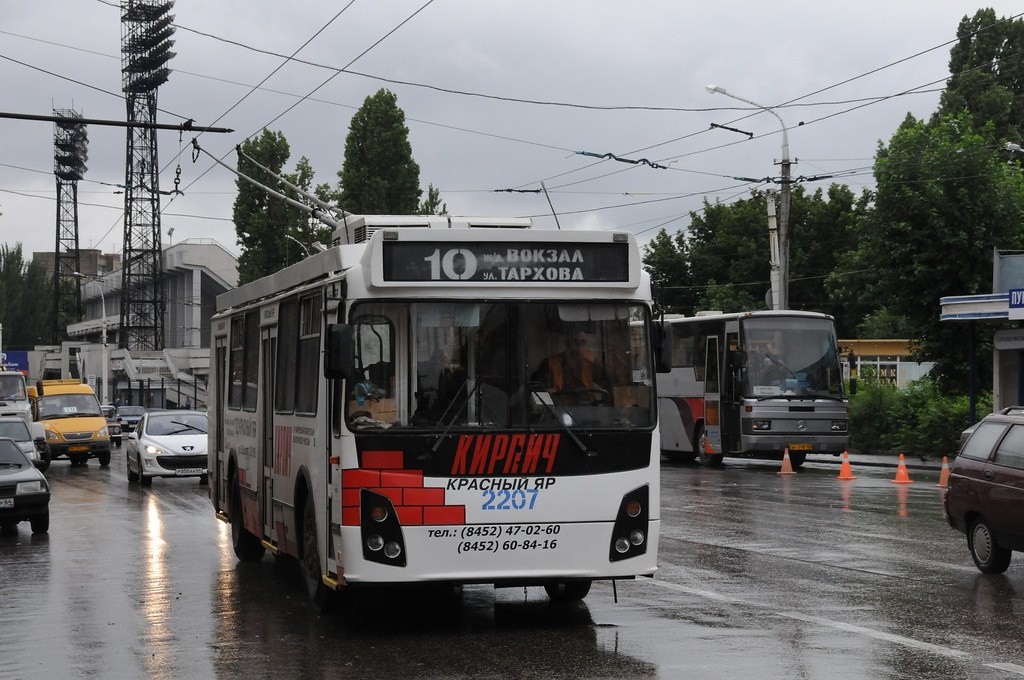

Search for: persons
xmin=429 ymin=347 xmax=459 ymax=384
xmin=534 ymin=328 xmax=619 ymax=408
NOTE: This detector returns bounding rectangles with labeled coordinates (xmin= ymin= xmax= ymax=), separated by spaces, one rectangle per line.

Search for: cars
xmin=0 ymin=412 xmax=46 ymax=469
xmin=0 ymin=437 xmax=50 ymax=532
xmin=128 ymin=411 xmax=210 ymax=487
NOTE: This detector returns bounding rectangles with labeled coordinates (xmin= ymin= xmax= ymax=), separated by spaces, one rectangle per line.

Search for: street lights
xmin=706 ymin=84 xmax=792 ymax=310
xmin=74 ymin=271 xmax=110 ymax=406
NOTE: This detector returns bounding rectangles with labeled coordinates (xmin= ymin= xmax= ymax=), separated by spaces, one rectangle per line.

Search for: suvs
xmin=942 ymin=405 xmax=1024 ymax=573
xmin=116 ymin=406 xmax=147 ymax=432
xmin=102 ymin=409 xmax=123 ymax=449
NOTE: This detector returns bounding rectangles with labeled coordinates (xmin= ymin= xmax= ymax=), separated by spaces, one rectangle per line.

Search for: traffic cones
xmin=935 ymin=456 xmax=952 ymax=488
xmin=777 ymin=449 xmax=796 ymax=476
xmin=890 ymin=454 xmax=913 ymax=484
xmin=835 ymin=452 xmax=857 ymax=479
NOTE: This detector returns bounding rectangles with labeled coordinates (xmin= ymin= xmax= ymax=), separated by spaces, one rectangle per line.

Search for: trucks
xmin=17 ymin=379 xmax=115 ymax=467
xmin=0 ymin=351 xmax=46 ymax=441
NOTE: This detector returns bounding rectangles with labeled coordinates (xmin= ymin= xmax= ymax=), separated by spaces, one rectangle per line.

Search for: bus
xmin=195 ymin=140 xmax=662 ymax=611
xmin=626 ymin=310 xmax=858 ymax=468
